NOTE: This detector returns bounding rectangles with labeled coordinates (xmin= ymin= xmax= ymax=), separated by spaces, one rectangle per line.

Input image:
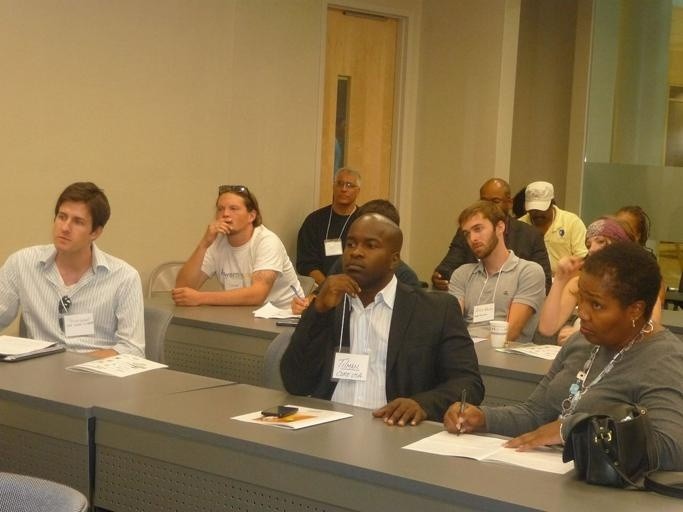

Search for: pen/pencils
xmin=457 ymin=389 xmax=466 ymax=436
xmin=290 ymin=285 xmax=304 ymax=302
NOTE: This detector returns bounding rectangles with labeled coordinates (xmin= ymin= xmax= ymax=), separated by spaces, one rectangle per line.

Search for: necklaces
xmin=558 ymin=319 xmax=654 ymax=424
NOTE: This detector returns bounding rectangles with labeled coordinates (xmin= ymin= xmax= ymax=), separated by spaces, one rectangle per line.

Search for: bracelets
xmin=560 ymin=422 xmax=568 ymax=447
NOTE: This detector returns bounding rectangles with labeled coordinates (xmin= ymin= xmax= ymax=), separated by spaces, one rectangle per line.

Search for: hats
xmin=524 ymin=180 xmax=556 ymax=211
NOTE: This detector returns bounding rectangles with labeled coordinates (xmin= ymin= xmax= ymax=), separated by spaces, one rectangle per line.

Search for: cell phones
xmin=262 ymin=404 xmax=299 ymax=418
xmin=438 ymin=266 xmax=449 ymax=283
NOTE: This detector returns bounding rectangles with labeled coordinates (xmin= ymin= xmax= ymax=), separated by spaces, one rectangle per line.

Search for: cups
xmin=490 ymin=320 xmax=508 ymax=348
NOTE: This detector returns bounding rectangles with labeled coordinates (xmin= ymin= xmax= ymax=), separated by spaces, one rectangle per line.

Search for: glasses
xmin=58 ymin=295 xmax=71 ymax=335
xmin=333 ymin=180 xmax=360 ymax=189
xmin=217 ymin=184 xmax=253 ymax=200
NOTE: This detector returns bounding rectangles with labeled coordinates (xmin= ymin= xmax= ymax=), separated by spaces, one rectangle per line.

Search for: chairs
xmin=148 ymin=263 xmax=209 ymax=294
xmin=299 ymin=275 xmax=315 ymax=297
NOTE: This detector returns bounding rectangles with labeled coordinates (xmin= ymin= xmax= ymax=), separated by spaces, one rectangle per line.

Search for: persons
xmin=614 ymin=206 xmax=656 ymax=258
xmin=280 ymin=212 xmax=485 ymax=427
xmin=538 ymin=217 xmax=666 ymax=342
xmin=0 ymin=181 xmax=145 ymax=361
xmin=433 ymin=177 xmax=552 ymax=295
xmin=445 ymin=241 xmax=682 ymax=469
xmin=328 ymin=198 xmax=419 ymax=285
xmin=516 ymin=181 xmax=589 ymax=268
xmin=172 ymin=185 xmax=305 ymax=309
xmin=296 ymin=169 xmax=360 ymax=284
xmin=448 ymin=201 xmax=546 ymax=344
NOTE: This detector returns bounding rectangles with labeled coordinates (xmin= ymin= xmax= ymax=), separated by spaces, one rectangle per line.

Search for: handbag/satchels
xmin=559 ymin=402 xmax=663 ymax=490
